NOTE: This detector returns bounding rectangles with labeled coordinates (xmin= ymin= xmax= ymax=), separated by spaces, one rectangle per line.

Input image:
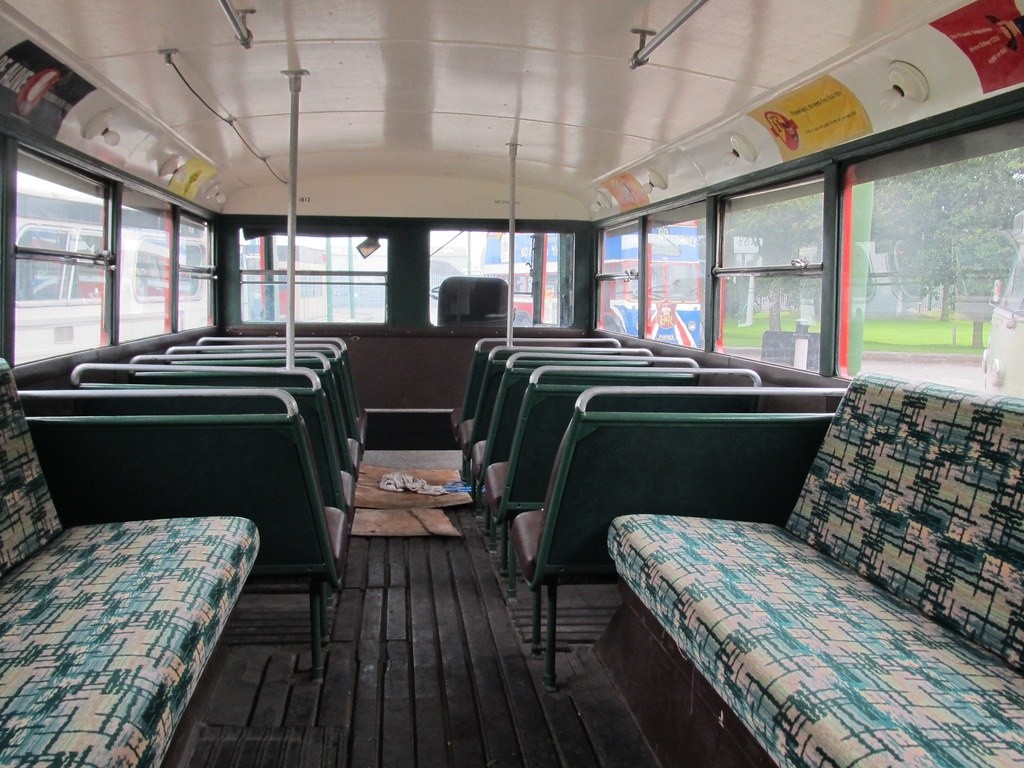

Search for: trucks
xmin=981 ymin=208 xmax=1024 ymax=400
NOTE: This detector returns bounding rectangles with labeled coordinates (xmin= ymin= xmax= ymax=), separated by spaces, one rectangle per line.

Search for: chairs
xmin=438 ymin=277 xmax=518 ymax=326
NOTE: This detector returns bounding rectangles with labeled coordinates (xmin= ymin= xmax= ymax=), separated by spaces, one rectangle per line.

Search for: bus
xmin=482 ymin=220 xmax=702 ymax=349
xmin=14 ymin=182 xmax=328 ymax=365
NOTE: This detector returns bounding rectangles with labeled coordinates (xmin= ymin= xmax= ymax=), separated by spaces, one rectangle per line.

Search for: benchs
xmin=17 ymin=336 xmax=369 ymax=684
xmin=608 ymin=369 xmax=1024 ymax=768
xmin=0 ymin=358 xmax=260 ymax=768
xmin=452 ymin=338 xmax=849 ymax=694
